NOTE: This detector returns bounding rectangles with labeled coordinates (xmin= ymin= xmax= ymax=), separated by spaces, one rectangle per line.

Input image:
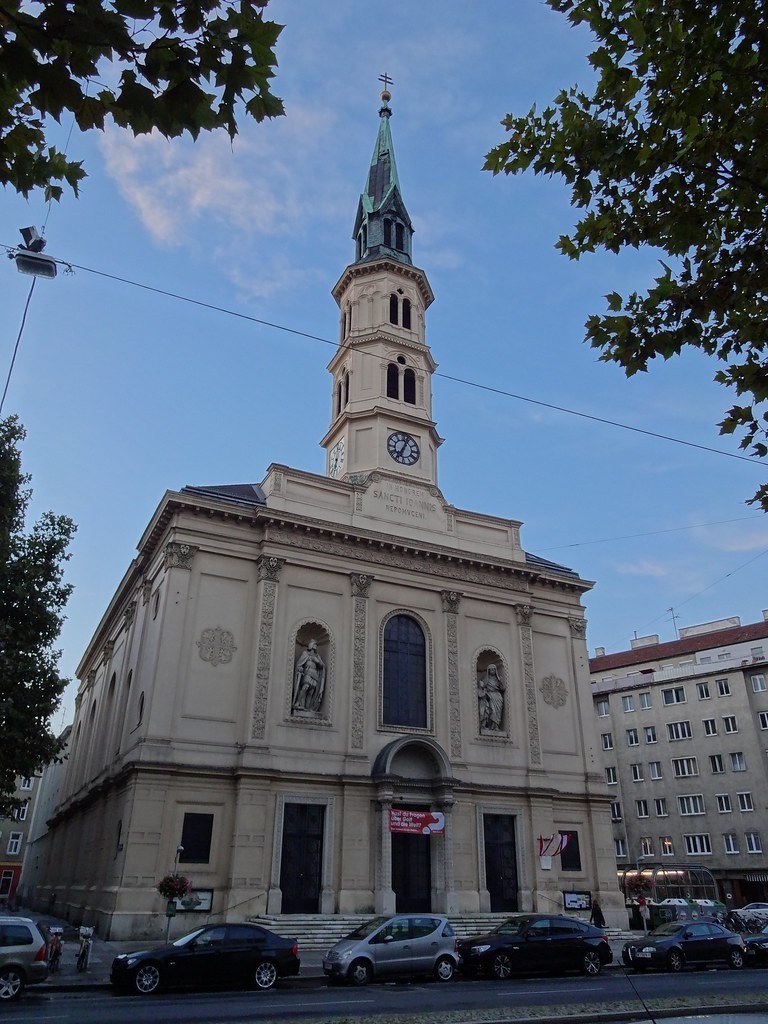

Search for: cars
xmin=322 ymin=912 xmax=461 ymax=986
xmin=742 ymin=923 xmax=768 ymax=969
xmin=458 ymin=913 xmax=614 ymax=981
xmin=731 ymin=901 xmax=768 ymax=922
xmin=109 ymin=921 xmax=300 ymax=995
xmin=623 ymin=921 xmax=748 ymax=975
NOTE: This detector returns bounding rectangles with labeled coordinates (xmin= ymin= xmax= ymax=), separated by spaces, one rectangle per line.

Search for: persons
xmin=589 ymin=899 xmax=605 ymax=929
xmin=479 ymin=664 xmax=506 ymax=732
xmin=292 ymin=638 xmax=327 ymax=712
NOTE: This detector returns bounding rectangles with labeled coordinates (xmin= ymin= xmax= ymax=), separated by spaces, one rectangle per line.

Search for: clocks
xmin=329 ymin=441 xmax=344 ymax=477
xmin=387 ymin=432 xmax=420 ymax=466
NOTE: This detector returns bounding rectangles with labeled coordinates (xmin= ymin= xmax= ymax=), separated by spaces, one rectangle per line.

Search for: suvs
xmin=0 ymin=916 xmax=48 ymax=1003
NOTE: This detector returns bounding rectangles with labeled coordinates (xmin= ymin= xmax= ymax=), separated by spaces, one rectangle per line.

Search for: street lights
xmin=635 ymin=856 xmax=648 ymax=937
xmin=164 ymin=843 xmax=184 ymax=945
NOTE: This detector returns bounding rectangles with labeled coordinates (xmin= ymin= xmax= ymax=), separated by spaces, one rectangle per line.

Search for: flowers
xmin=626 ymin=875 xmax=656 ymax=894
xmin=156 ymin=875 xmax=192 ymax=900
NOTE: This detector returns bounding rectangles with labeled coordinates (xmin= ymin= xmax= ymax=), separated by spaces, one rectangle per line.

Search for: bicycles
xmin=712 ymin=910 xmax=768 ymax=934
xmin=47 ymin=925 xmax=67 ymax=976
xmin=71 ymin=926 xmax=94 ymax=973
